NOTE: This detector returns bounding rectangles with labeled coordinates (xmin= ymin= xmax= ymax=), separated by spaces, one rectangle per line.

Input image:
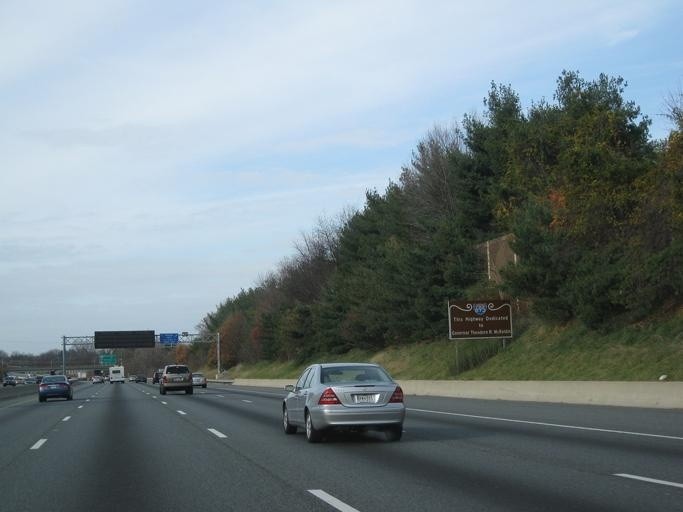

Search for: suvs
xmin=159 ymin=362 xmax=195 ymax=396
xmin=150 ymin=368 xmax=164 ymax=384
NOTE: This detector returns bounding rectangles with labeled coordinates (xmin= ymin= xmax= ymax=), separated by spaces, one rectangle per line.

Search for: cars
xmin=190 ymin=372 xmax=208 ymax=388
xmin=278 ymin=359 xmax=410 ymax=443
xmin=89 ymin=372 xmax=147 ymax=384
xmin=36 ymin=373 xmax=73 ymax=402
xmin=3 ymin=373 xmax=46 ymax=386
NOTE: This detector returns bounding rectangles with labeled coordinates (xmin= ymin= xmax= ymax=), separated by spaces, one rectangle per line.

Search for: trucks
xmin=107 ymin=365 xmax=124 ymax=384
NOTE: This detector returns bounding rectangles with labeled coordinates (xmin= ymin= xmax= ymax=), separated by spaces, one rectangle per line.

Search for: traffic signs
xmin=159 ymin=333 xmax=178 ymax=343
xmin=98 ymin=354 xmax=117 ymax=367
